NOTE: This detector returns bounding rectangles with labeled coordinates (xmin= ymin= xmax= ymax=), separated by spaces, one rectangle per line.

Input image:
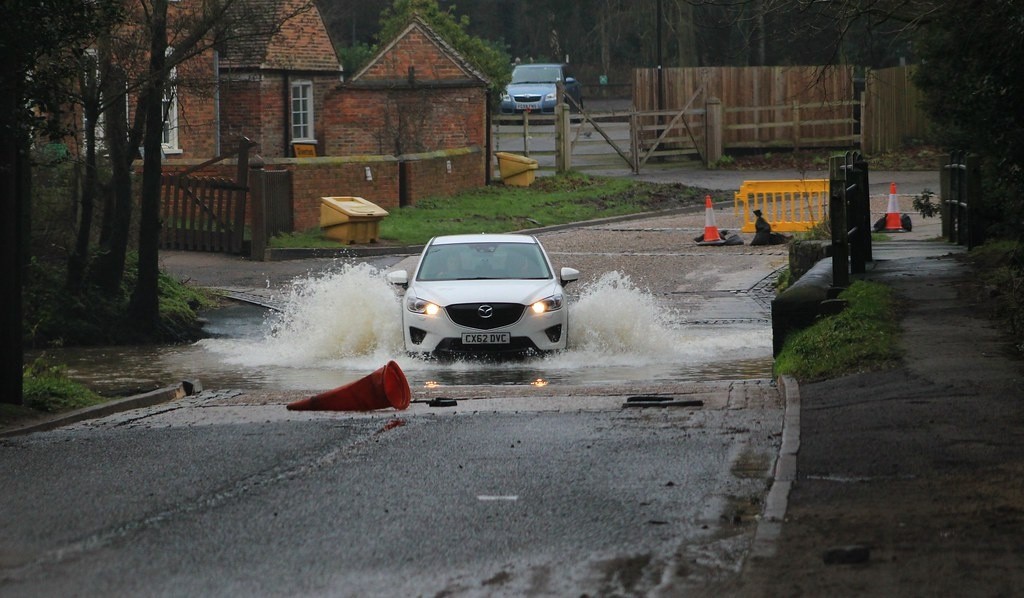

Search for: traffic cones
xmin=286 ymin=360 xmax=413 ymax=411
xmin=694 ymin=194 xmax=726 ymax=246
xmin=875 ymin=182 xmax=909 ymax=234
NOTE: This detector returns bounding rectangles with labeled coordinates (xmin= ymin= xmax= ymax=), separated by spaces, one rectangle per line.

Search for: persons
xmin=439 ymin=250 xmax=468 ymax=277
xmin=507 ymin=252 xmax=529 ymax=275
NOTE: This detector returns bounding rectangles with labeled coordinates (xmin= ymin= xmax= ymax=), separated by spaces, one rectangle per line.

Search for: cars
xmin=387 ymin=233 xmax=580 ymax=358
xmin=498 ymin=63 xmax=584 ymax=113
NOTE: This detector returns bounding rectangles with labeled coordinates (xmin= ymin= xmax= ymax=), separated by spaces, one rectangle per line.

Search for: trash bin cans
xmin=318 ymin=194 xmax=389 ymax=245
xmin=495 ymin=152 xmax=540 ymax=188
